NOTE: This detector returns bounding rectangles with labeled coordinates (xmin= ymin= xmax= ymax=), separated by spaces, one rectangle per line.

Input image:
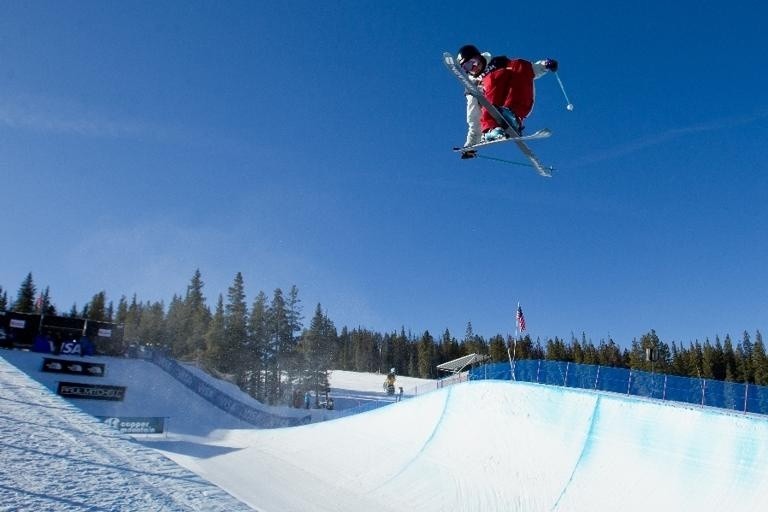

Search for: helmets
xmin=457 ymin=45 xmax=481 ymax=65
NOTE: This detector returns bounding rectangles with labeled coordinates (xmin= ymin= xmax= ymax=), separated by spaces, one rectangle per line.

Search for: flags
xmin=516 ymin=306 xmax=526 ymax=332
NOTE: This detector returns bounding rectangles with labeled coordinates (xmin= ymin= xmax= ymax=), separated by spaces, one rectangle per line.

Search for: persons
xmin=456 ymin=44 xmax=557 ymax=159
xmin=293 ymin=388 xmax=333 ymax=410
xmin=384 ymin=373 xmax=395 ymax=394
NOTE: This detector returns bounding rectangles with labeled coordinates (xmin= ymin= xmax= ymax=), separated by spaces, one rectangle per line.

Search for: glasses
xmin=462 ymin=56 xmax=480 ymax=71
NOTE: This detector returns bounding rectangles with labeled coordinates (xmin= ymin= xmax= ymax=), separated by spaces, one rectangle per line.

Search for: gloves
xmin=462 ymin=150 xmax=477 ymax=160
xmin=545 ymin=60 xmax=557 ymax=72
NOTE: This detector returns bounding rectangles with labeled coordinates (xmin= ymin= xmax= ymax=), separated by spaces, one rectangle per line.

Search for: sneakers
xmin=501 ymin=105 xmax=521 ymax=130
xmin=485 ymin=127 xmax=506 ymax=142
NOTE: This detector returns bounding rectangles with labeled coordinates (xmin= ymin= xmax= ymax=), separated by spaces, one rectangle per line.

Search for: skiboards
xmin=442 ymin=52 xmax=554 ymax=177
